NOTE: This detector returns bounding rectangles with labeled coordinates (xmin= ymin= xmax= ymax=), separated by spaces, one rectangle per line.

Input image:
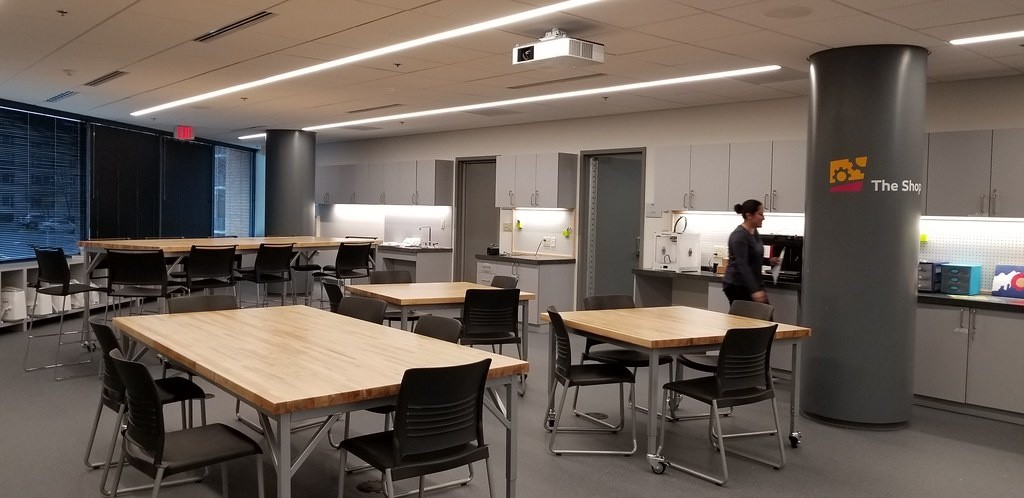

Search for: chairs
xmin=23 ymin=233 xmax=786 ymax=498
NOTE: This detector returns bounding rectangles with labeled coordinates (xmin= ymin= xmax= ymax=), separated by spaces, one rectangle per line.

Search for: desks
xmin=343 ymin=281 xmax=536 ymax=384
xmin=916 ymin=289 xmax=1024 ymax=315
xmin=538 ymin=305 xmax=812 ymax=475
xmin=75 ymin=235 xmax=384 ymax=352
xmin=112 ymin=304 xmax=530 ymax=498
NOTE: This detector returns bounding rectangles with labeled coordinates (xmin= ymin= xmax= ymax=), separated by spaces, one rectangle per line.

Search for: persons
xmin=722 ymin=200 xmax=774 ymax=322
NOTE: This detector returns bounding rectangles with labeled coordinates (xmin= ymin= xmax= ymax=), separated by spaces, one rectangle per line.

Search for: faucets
xmin=419 ymin=225 xmax=438 ymax=247
xmin=535 ymin=239 xmax=546 ymax=257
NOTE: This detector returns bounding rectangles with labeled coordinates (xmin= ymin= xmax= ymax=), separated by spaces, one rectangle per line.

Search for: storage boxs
xmin=917 ymin=262 xmax=981 ymax=296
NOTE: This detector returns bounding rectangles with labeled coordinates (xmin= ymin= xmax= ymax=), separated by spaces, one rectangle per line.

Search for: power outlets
xmin=542 ymin=237 xmax=551 ymax=247
xmin=713 ymin=246 xmax=726 ymax=258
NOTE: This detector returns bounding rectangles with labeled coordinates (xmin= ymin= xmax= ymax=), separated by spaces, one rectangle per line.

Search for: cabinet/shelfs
xmin=319 ymin=159 xmax=454 ymax=205
xmin=477 ymin=262 xmax=576 ymax=325
xmin=0 ymin=252 xmax=185 ymax=334
xmin=654 ymin=127 xmax=1024 ymax=218
xmin=495 ymin=153 xmax=577 ymax=209
xmin=914 ymin=303 xmax=1024 ymax=415
xmin=707 ymin=282 xmax=797 ymax=371
